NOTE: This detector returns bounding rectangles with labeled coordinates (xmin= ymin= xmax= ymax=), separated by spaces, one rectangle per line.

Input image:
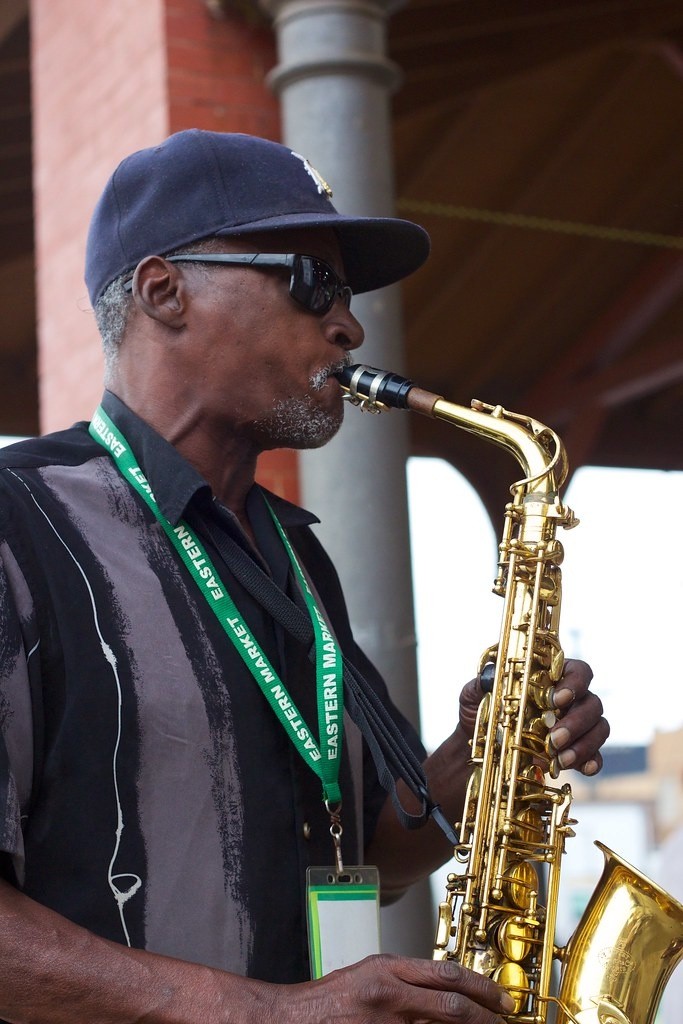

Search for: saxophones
xmin=339 ymin=355 xmax=683 ymax=1024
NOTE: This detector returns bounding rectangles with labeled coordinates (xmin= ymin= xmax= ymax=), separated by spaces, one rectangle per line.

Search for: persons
xmin=0 ymin=129 xmax=611 ymax=1024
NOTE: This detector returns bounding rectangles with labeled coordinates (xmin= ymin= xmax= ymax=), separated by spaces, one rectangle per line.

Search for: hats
xmin=81 ymin=128 xmax=433 ymax=309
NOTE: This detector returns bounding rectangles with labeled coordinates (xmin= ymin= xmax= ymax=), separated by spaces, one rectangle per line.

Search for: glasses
xmin=120 ymin=246 xmax=354 ymax=318
